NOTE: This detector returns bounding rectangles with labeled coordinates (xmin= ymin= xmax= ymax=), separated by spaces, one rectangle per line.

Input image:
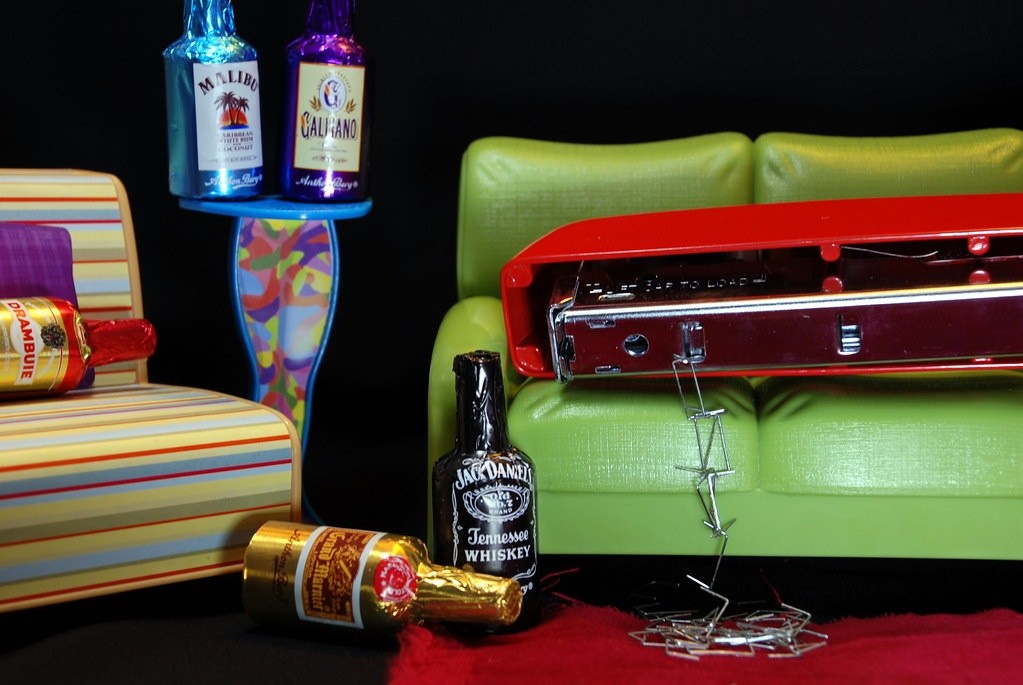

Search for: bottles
xmin=161 ymin=0 xmax=264 ymax=201
xmin=244 ymin=519 xmax=524 ymax=635
xmin=431 ymin=350 xmax=543 ymax=635
xmin=0 ymin=295 xmax=157 ymax=399
xmin=280 ymin=0 xmax=369 ymax=203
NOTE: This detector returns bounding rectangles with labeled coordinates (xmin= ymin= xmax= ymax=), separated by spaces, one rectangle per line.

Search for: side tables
xmin=176 ymin=191 xmax=374 ymax=527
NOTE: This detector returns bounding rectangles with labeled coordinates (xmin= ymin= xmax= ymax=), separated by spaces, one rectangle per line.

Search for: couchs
xmin=0 ymin=169 xmax=300 ymax=615
xmin=426 ymin=127 xmax=1023 ymax=578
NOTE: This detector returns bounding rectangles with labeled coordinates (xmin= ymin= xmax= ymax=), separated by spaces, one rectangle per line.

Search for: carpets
xmin=385 ymin=590 xmax=1023 ymax=685
